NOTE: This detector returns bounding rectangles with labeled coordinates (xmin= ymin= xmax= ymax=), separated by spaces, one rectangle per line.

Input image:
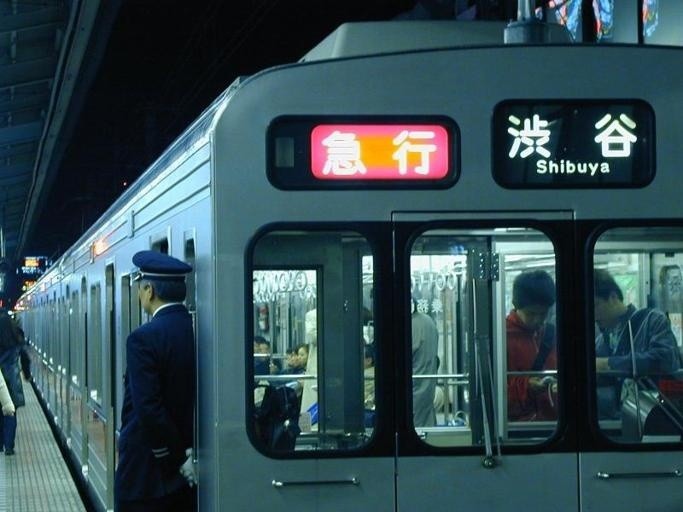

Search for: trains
xmin=14 ymin=0 xmax=683 ymax=511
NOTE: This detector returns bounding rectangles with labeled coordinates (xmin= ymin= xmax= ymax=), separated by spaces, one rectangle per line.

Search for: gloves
xmin=179 ymin=448 xmax=197 ymax=487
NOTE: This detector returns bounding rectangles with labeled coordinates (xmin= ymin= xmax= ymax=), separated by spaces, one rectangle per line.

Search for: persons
xmin=0 ymin=307 xmax=31 ymax=455
xmin=593 ymin=267 xmax=678 ymax=419
xmin=254 ymin=307 xmax=375 ymax=428
xmin=115 ymin=250 xmax=196 ymax=512
xmin=0 ymin=370 xmax=15 ymax=416
xmin=411 ymin=298 xmax=439 ymax=428
xmin=506 ymin=270 xmax=558 ymax=437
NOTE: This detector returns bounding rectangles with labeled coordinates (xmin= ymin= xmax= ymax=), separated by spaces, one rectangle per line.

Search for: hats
xmin=132 ymin=251 xmax=192 ymax=282
